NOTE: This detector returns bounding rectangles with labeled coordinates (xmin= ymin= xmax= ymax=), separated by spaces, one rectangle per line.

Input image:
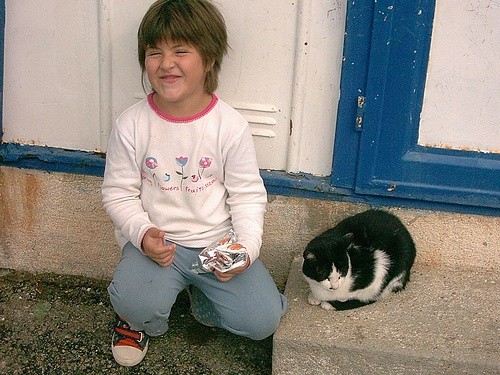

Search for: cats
xmin=301 ymin=208 xmax=416 ymax=311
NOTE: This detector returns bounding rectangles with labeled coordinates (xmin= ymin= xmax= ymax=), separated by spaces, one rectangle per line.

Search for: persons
xmin=100 ymin=1 xmax=287 ymax=366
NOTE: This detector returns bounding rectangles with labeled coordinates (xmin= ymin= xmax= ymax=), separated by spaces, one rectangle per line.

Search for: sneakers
xmin=112 ymin=314 xmax=149 ymax=366
